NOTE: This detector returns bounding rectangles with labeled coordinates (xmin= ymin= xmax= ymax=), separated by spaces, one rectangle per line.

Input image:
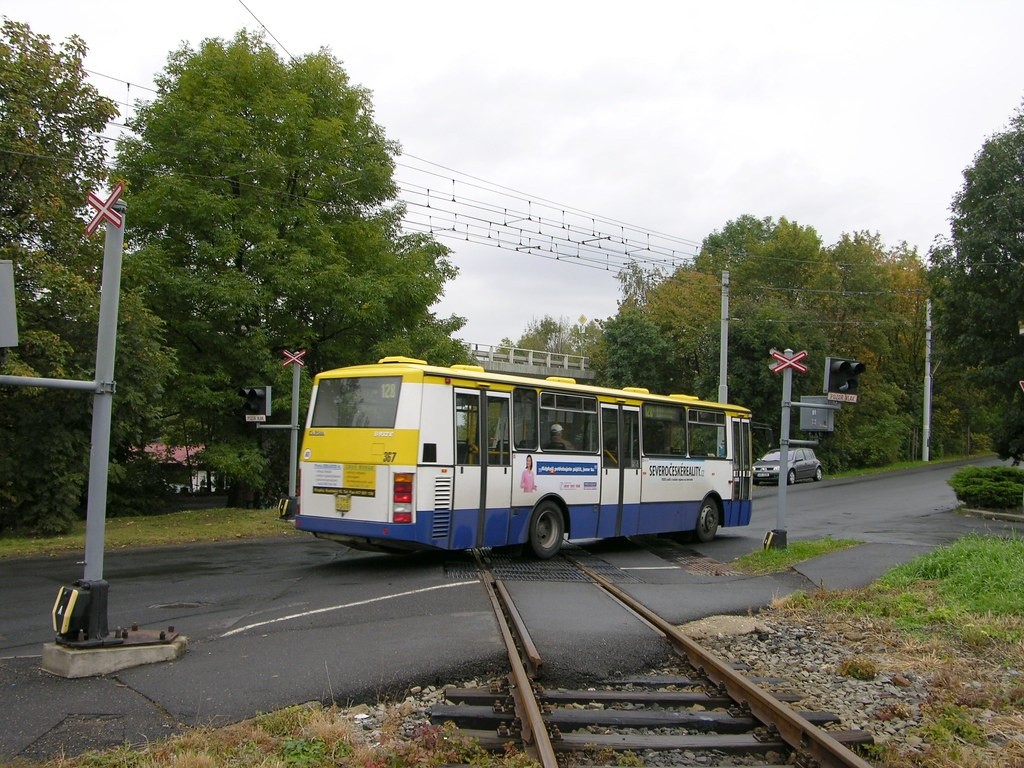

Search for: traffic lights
xmin=233 ymin=385 xmax=272 ymax=422
xmin=823 ymin=357 xmax=866 ymax=403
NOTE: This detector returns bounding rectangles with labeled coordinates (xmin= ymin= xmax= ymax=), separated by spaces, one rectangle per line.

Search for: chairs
xmin=457 ymin=440 xmax=471 ymax=464
xmin=495 ymin=440 xmax=517 ymax=464
xmin=545 ymin=442 xmax=567 ymax=452
xmin=519 ymin=440 xmax=536 ymax=453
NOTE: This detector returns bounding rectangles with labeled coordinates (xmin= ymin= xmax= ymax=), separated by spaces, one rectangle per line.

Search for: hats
xmin=551 ymin=424 xmax=564 ymax=432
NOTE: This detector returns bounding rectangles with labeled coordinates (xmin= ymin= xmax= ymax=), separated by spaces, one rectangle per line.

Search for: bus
xmin=296 ymin=356 xmax=752 ymax=559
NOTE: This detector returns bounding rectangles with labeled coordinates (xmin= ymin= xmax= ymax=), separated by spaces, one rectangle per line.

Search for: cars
xmin=752 ymin=448 xmax=822 ymax=485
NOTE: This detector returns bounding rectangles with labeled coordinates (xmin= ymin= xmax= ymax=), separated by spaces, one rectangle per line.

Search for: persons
xmin=550 ymin=424 xmax=575 ymax=449
xmin=519 ymin=455 xmax=538 ymax=492
